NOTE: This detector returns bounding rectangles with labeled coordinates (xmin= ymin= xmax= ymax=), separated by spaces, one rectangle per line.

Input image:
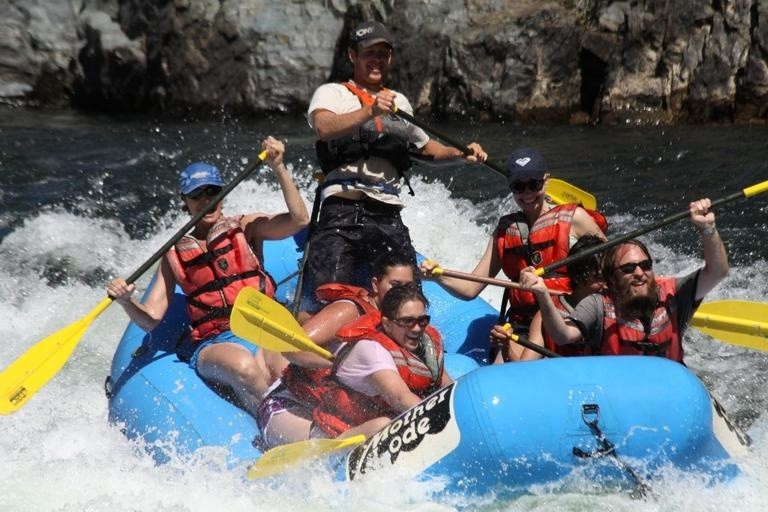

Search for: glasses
xmin=510 ymin=179 xmax=543 ymax=194
xmin=614 ymin=260 xmax=651 ymax=272
xmin=392 ymin=315 xmax=430 ymax=328
xmin=187 ymin=185 xmax=223 ymax=199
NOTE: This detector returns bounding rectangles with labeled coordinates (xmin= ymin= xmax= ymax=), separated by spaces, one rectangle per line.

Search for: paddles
xmin=427 ymin=257 xmax=768 ymax=352
xmin=390 ymin=103 xmax=596 ymax=211
xmin=248 ymin=431 xmax=368 ymax=476
xmin=230 ymin=286 xmax=337 ymax=367
xmin=1 ymin=139 xmax=272 ymax=415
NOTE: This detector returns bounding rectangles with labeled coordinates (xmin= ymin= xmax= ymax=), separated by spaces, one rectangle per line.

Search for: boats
xmin=103 ymin=211 xmax=740 ymax=506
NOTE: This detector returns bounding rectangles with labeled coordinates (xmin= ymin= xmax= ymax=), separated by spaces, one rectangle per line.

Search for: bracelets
xmin=702 ymin=223 xmax=717 ymax=238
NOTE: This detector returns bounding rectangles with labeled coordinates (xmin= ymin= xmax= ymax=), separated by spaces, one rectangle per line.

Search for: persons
xmin=255 ymin=251 xmax=423 ymax=449
xmin=294 ymin=20 xmax=488 ymax=327
xmin=519 ymin=197 xmax=731 ymax=361
xmin=416 ymin=147 xmax=608 ymax=364
xmin=105 ymin=133 xmax=310 ymax=417
xmin=310 ymin=281 xmax=454 ymax=443
xmin=488 ymin=232 xmax=609 ymax=362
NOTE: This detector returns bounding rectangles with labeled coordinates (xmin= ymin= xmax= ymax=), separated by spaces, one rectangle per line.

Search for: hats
xmin=506 ymin=149 xmax=545 ymax=184
xmin=350 ymin=22 xmax=392 ymax=49
xmin=180 ymin=162 xmax=225 ymax=195
